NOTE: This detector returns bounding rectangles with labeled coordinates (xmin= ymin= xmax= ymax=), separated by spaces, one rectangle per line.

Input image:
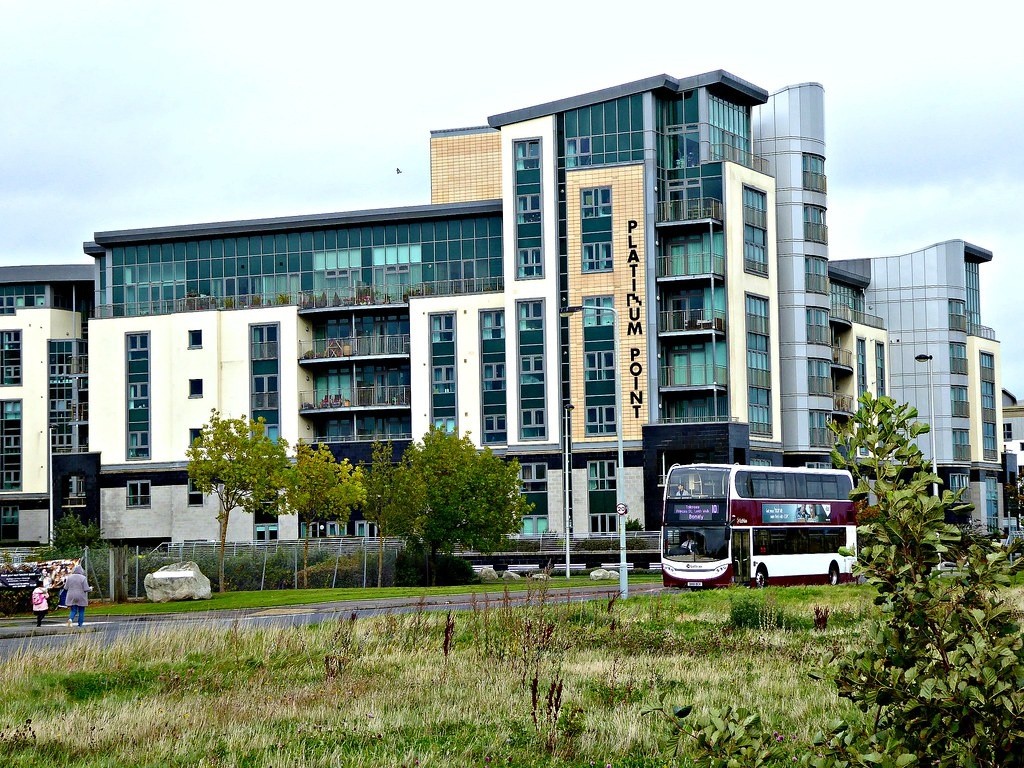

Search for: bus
xmin=659 ymin=462 xmax=860 ymax=589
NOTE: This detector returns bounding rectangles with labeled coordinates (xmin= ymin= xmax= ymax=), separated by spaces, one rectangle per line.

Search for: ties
xmin=686 ymin=539 xmax=690 ymax=549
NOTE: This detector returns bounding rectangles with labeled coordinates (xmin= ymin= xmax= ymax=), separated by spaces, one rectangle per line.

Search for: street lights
xmin=915 ymin=355 xmax=940 ymax=499
xmin=563 ymin=403 xmax=577 ymax=577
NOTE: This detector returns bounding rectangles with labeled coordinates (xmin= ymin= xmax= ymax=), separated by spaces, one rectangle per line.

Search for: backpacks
xmin=32 ymin=591 xmax=46 ymax=606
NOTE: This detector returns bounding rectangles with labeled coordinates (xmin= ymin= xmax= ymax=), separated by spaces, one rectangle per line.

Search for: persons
xmin=797 ymin=504 xmax=819 ymax=523
xmin=32 ymin=561 xmax=94 ymax=629
xmin=675 ymin=483 xmax=690 ymax=496
xmin=679 ymin=532 xmax=699 ymax=553
